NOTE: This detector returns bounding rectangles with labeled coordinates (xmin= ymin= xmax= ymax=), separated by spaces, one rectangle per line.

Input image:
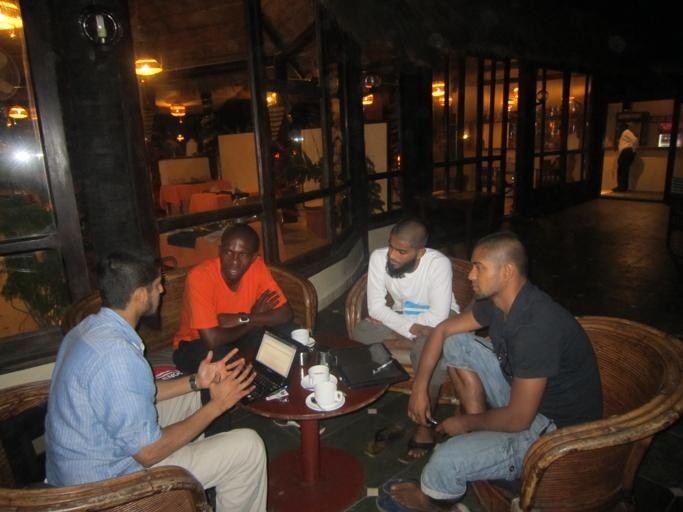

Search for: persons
xmin=45 ymin=244 xmax=268 ymax=512
xmin=354 ymin=219 xmax=461 ymax=465
xmin=173 ymin=222 xmax=296 ymax=373
xmin=612 ymin=121 xmax=639 ymax=193
xmin=390 ymin=228 xmax=603 ymax=511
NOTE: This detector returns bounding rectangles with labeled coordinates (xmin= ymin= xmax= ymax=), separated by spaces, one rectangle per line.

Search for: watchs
xmin=235 ymin=312 xmax=250 ymax=324
xmin=188 ymin=373 xmax=199 ymax=390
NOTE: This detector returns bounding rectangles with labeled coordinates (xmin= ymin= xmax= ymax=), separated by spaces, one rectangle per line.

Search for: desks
xmin=220 ymin=334 xmax=387 ymax=512
xmin=160 ymin=180 xmax=233 ymax=215
xmin=190 ymin=189 xmax=259 ymax=213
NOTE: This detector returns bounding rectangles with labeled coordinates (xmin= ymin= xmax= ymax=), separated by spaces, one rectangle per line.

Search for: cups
xmin=308 ymin=364 xmax=330 ymax=385
xmin=290 ymin=328 xmax=309 ymax=345
xmin=315 ymin=383 xmax=343 ymax=406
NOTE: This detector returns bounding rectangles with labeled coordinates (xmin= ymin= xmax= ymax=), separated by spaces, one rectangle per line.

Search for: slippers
xmin=363 ymin=428 xmax=401 ymax=456
xmin=374 ymin=492 xmax=467 ymax=512
xmin=399 ymin=434 xmax=435 ymax=465
xmin=382 ymin=477 xmax=419 ymax=494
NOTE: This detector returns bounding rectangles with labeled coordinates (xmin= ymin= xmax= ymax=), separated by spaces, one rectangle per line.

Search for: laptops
xmin=234 ymin=325 xmax=304 ymax=406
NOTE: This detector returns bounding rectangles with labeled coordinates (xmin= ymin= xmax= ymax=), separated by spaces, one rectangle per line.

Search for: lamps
xmin=136 ymin=50 xmax=161 ymax=77
xmin=9 ymin=103 xmax=27 ymax=118
xmin=0 ymin=1 xmax=24 ymax=29
xmin=170 ymin=101 xmax=185 ymax=117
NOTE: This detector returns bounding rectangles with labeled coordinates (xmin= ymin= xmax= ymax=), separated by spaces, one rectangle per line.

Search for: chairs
xmin=0 ymin=377 xmax=211 ymax=512
xmin=456 ymin=315 xmax=683 ymax=511
xmin=59 ymin=262 xmax=317 ymax=399
xmin=347 ymin=257 xmax=476 ymax=425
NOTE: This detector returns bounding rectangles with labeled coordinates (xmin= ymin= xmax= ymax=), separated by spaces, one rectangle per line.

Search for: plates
xmin=304 ymin=337 xmax=315 ymax=347
xmin=305 ymin=391 xmax=345 ymax=412
xmin=300 ymin=374 xmax=338 ymax=392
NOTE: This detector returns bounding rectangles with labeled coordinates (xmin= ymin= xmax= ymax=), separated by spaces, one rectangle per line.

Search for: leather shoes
xmin=611 ymin=187 xmax=626 ymax=193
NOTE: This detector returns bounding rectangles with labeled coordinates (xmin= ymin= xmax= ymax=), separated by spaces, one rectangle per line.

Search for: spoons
xmin=309 ymin=397 xmax=325 ymax=412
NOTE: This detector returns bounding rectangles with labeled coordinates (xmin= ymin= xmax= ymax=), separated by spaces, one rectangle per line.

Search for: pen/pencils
xmin=371 ymin=360 xmax=393 ymax=374
xmin=427 ymin=417 xmax=438 ymax=424
